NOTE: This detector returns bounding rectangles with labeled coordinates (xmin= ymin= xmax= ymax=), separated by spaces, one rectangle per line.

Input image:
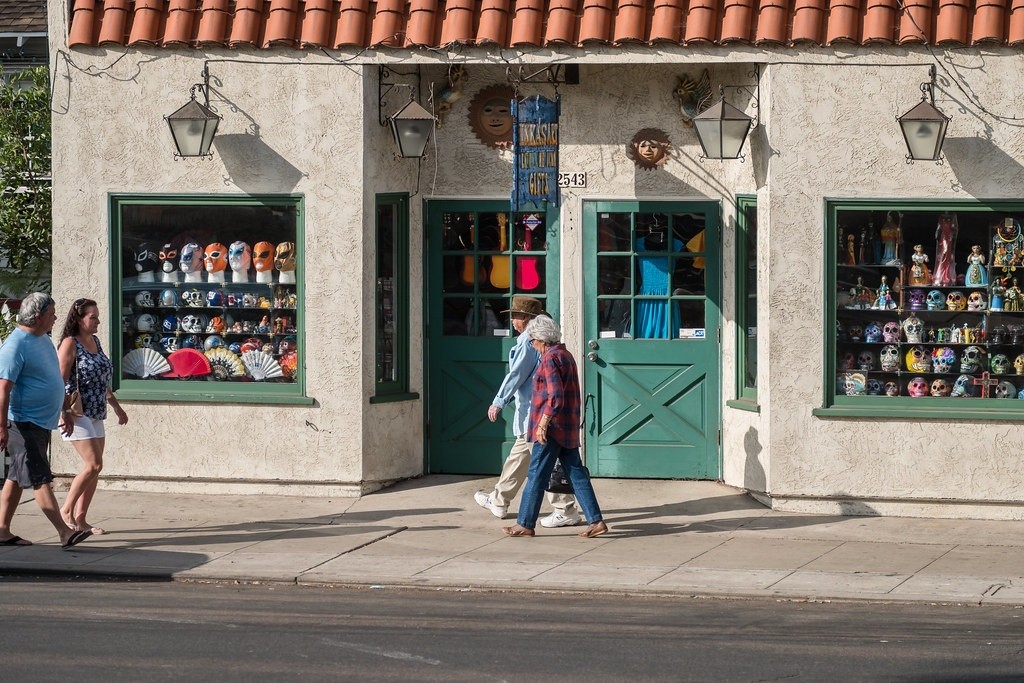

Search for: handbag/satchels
xmin=545 ymin=463 xmax=590 ymax=494
xmin=53 ymin=336 xmax=83 ymax=417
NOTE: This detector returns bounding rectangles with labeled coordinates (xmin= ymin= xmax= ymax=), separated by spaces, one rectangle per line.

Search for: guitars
xmin=488 ymin=212 xmax=512 ymax=289
xmin=460 ymin=210 xmax=487 ymax=285
xmin=514 ymin=213 xmax=542 ymax=291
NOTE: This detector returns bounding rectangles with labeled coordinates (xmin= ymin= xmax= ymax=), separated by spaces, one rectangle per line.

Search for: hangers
xmin=643 ymin=212 xmax=667 ymax=241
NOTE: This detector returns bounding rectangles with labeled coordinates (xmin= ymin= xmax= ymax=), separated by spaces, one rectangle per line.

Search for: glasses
xmin=74 ymin=299 xmax=83 ymax=310
xmin=529 ymin=339 xmax=536 ymax=344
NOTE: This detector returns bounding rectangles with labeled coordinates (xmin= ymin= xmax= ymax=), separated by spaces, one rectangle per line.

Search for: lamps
xmin=162 ymin=62 xmax=224 ymax=161
xmin=379 ymin=64 xmax=441 ymax=162
xmin=692 ymin=64 xmax=759 ymax=163
xmin=895 ymin=65 xmax=952 ymax=166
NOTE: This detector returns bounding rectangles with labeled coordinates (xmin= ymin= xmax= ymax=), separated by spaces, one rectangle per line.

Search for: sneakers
xmin=540 ymin=509 xmax=581 ymax=527
xmin=474 ymin=491 xmax=507 ymax=518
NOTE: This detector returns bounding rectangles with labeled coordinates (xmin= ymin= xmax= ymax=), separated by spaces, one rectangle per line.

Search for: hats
xmin=500 ymin=297 xmax=553 ymax=319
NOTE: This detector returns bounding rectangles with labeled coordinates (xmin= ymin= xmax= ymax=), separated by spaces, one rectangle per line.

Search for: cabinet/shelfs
xmin=121 ymin=282 xmax=299 ymax=357
xmin=834 ymin=222 xmax=1024 ymax=397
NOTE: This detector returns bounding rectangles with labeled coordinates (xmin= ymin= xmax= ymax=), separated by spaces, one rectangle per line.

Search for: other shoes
xmin=580 ymin=520 xmax=608 ymax=537
xmin=58 ymin=508 xmax=82 ymax=532
xmin=76 ymin=521 xmax=106 ymax=534
xmin=500 ymin=524 xmax=535 ymax=537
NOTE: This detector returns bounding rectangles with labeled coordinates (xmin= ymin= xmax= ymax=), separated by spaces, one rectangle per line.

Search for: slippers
xmin=61 ymin=531 xmax=92 ymax=550
xmin=0 ymin=536 xmax=32 ymax=546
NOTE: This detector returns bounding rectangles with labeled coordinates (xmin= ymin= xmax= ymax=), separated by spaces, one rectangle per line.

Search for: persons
xmin=0 ymin=292 xmax=93 ymax=550
xmin=253 ymin=241 xmax=274 ymax=283
xmin=847 ymin=235 xmax=855 ymax=264
xmin=179 ymin=243 xmax=204 ymax=282
xmin=274 ymin=242 xmax=296 ymax=283
xmin=502 ymin=314 xmax=608 ymax=538
xmin=57 ymin=299 xmax=128 ymax=534
xmin=850 ymin=275 xmax=896 ymax=309
xmin=964 ymin=244 xmax=988 ymax=286
xmin=880 ymin=216 xmax=900 ymax=259
xmin=858 ymin=223 xmax=876 ymax=265
xmin=932 ymin=211 xmax=958 ymax=286
xmin=203 ymin=243 xmax=228 ymax=283
xmin=991 ymin=277 xmax=1021 ymax=311
xmin=134 ymin=241 xmax=158 ymax=282
xmin=229 ymin=241 xmax=250 ymax=283
xmin=158 ymin=241 xmax=181 ymax=283
xmin=474 ymin=296 xmax=581 ymax=527
xmin=908 ymin=244 xmax=932 ymax=286
